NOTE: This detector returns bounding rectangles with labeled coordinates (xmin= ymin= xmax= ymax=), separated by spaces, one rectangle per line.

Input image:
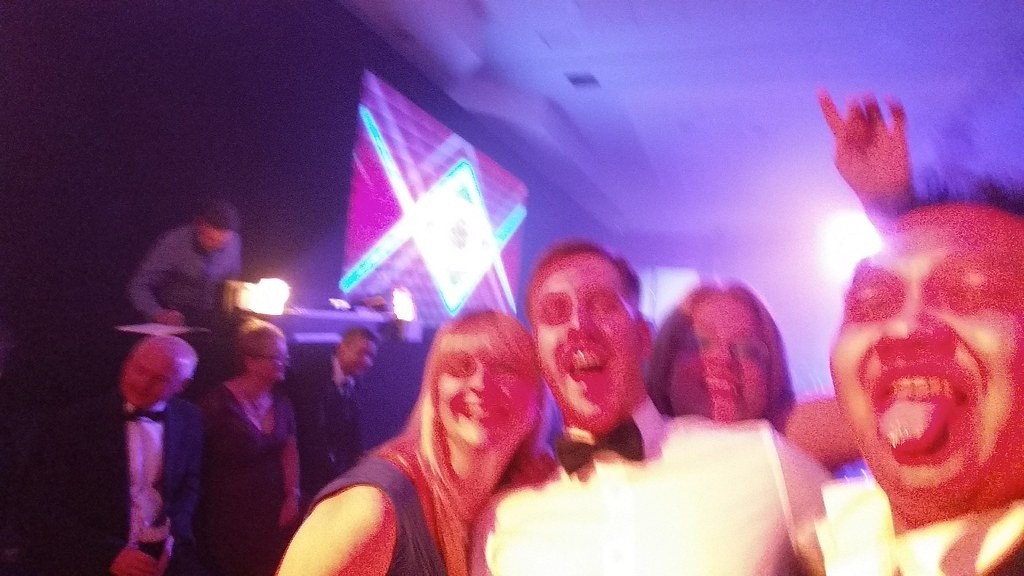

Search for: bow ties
xmin=124 ymin=408 xmax=165 ymax=423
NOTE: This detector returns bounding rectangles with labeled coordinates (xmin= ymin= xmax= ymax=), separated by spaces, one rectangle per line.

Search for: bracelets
xmin=283 ymin=486 xmax=301 ymax=496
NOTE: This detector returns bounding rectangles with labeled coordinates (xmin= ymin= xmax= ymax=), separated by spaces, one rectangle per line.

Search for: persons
xmin=467 ymin=236 xmax=832 ymax=576
xmin=13 ymin=332 xmax=207 ymax=576
xmin=812 ymin=158 xmax=1024 ymax=576
xmin=123 ymin=199 xmax=241 ymax=386
xmin=648 ymin=80 xmax=919 ymax=469
xmin=190 ymin=318 xmax=314 ymax=576
xmin=287 ymin=327 xmax=380 ymax=500
xmin=274 ymin=308 xmax=557 ymax=576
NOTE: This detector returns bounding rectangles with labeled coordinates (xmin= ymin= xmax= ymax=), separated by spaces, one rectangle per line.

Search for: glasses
xmin=245 ymin=351 xmax=289 ymax=366
